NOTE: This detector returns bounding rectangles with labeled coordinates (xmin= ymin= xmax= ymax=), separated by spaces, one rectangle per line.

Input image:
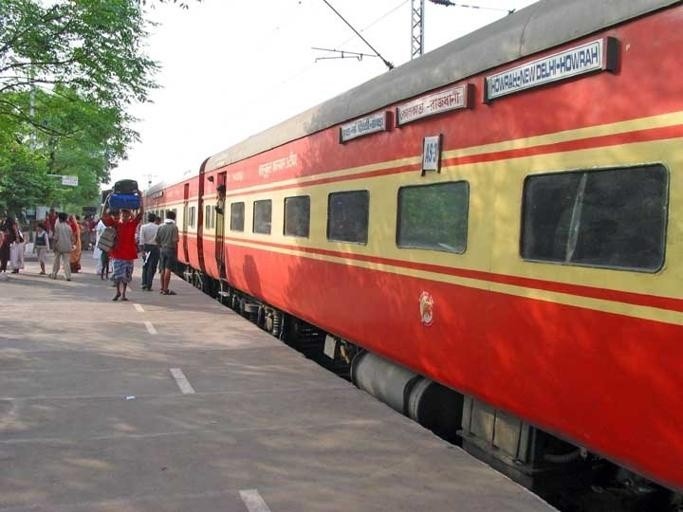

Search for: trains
xmin=139 ymin=1 xmax=683 ymax=512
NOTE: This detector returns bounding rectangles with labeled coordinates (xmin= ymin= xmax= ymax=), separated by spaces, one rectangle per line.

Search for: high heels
xmin=1 ymin=268 xmax=7 ymax=274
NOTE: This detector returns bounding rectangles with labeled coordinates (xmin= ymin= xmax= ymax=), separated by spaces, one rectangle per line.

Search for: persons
xmin=0 ymin=189 xmax=179 ymax=301
xmin=214 ymin=184 xmax=225 ymax=213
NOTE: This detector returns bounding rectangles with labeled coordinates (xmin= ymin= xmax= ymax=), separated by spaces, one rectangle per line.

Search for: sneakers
xmin=140 ymin=284 xmax=153 ymax=292
xmin=112 ymin=292 xmax=127 ymax=302
xmin=11 ymin=268 xmax=20 ymax=274
xmin=159 ymin=287 xmax=176 ymax=296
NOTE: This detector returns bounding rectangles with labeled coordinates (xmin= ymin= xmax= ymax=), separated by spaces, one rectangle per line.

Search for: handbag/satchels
xmin=96 ymin=227 xmax=116 ymax=252
xmin=9 ymin=240 xmax=27 ymax=269
xmin=107 ymin=179 xmax=141 ymax=210
